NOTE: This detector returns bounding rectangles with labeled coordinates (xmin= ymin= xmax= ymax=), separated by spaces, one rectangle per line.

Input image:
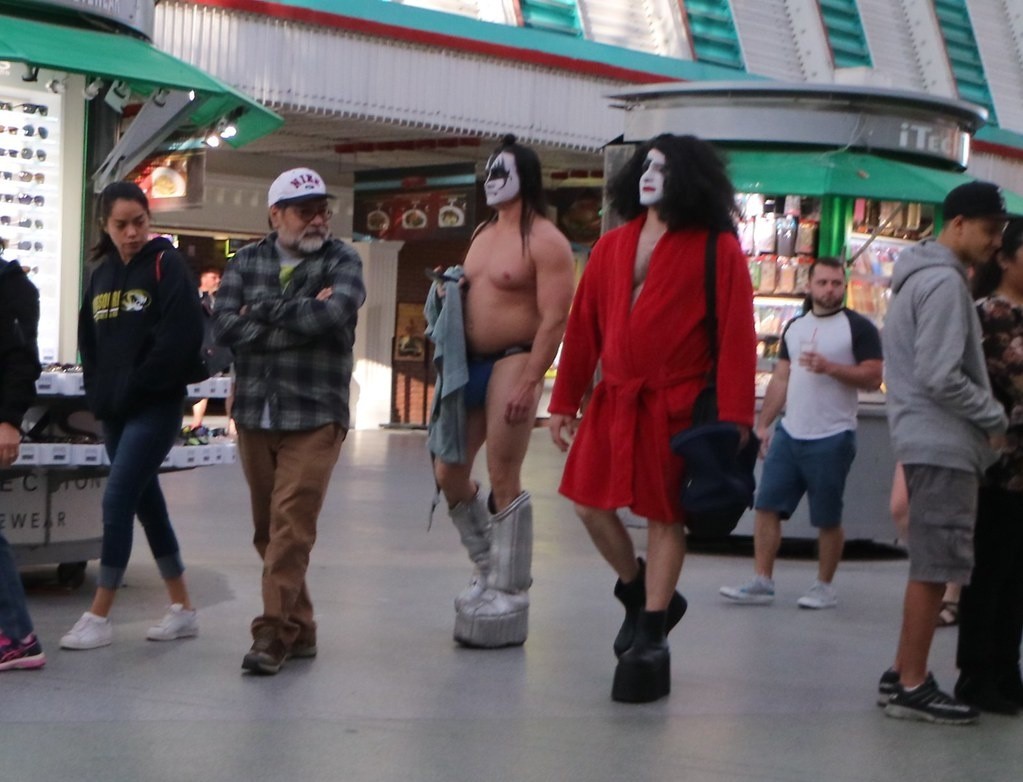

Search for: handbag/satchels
xmin=156 ymin=251 xmax=235 ymax=384
xmin=889 ymin=463 xmax=912 ymax=534
xmin=671 ymin=419 xmax=763 ymax=541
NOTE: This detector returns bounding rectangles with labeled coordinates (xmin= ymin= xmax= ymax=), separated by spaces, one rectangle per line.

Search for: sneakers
xmin=241 ymin=634 xmax=291 ymax=674
xmin=877 ymin=664 xmax=901 ymax=706
xmin=287 ymin=644 xmax=319 ymax=659
xmin=59 ymin=611 xmax=114 ymax=648
xmin=2 ymin=630 xmax=46 ymax=672
xmin=883 ymin=670 xmax=980 ymax=725
xmin=145 ymin=601 xmax=200 ymax=641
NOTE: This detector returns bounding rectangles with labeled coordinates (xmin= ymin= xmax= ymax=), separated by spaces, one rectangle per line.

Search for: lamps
xmin=21 ymin=64 xmax=245 ymax=150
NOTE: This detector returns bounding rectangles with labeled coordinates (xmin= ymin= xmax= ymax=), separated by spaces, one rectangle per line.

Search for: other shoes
xmin=796 ymin=582 xmax=838 ymax=608
xmin=719 ymin=578 xmax=775 ymax=602
xmin=934 ymin=600 xmax=958 ymax=626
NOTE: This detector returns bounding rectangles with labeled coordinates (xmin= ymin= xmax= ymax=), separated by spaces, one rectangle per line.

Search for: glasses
xmin=291 ymin=200 xmax=334 ymax=221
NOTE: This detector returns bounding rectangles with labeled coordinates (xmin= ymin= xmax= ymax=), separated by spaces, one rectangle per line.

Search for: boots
xmin=454 ymin=489 xmax=532 ymax=648
xmin=614 ymin=555 xmax=690 ymax=659
xmin=612 ymin=611 xmax=670 ymax=702
xmin=449 ymin=481 xmax=496 ymax=613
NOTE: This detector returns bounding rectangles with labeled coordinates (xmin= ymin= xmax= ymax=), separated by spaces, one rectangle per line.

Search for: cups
xmin=799 ymin=339 xmax=818 ymax=355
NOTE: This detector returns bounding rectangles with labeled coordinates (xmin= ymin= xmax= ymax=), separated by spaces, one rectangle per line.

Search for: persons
xmin=550 ymin=133 xmax=758 ymax=704
xmin=0 ymin=240 xmax=47 ymax=671
xmin=720 ymin=256 xmax=883 ymax=609
xmin=214 ymin=167 xmax=367 ymax=674
xmin=877 ymin=181 xmax=1023 ymax=724
xmin=61 ymin=181 xmax=208 ymax=650
xmin=423 ymin=135 xmax=574 ymax=646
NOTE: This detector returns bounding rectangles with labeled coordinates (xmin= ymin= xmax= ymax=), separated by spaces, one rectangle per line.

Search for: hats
xmin=268 ymin=167 xmax=336 ymax=207
xmin=944 ymin=179 xmax=1023 ymax=220
